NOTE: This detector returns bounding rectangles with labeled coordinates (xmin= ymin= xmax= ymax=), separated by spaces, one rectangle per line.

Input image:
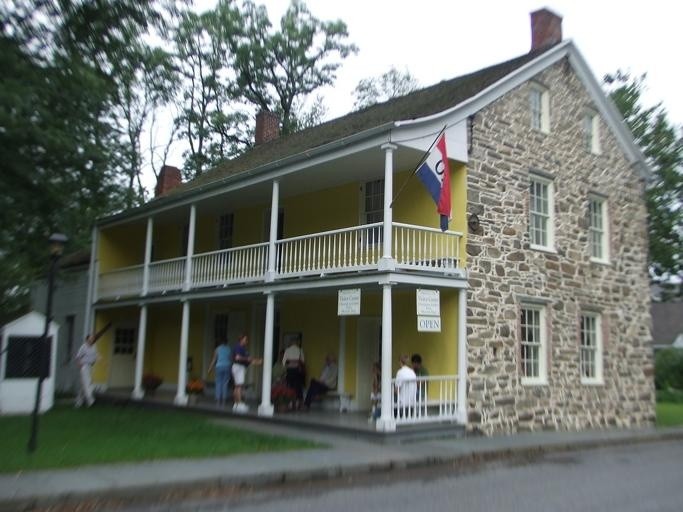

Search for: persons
xmin=369 ymin=353 xmax=430 ymax=422
xmin=73 ymin=333 xmax=99 ymax=410
xmin=187 ymin=356 xmax=207 ymax=404
xmin=270 ymin=335 xmax=337 ymax=414
xmin=232 ymin=333 xmax=262 ymax=411
xmin=207 ymin=331 xmax=233 ymax=407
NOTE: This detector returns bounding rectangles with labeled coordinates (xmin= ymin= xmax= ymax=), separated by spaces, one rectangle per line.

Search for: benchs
xmin=392 ymin=400 xmax=454 ymax=409
xmin=325 ymin=392 xmax=353 ymax=413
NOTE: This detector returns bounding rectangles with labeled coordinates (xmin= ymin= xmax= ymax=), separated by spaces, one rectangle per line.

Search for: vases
xmin=190 ymin=394 xmax=199 ymax=404
xmin=278 ymin=403 xmax=288 ymax=412
xmin=147 ymin=390 xmax=154 ymax=398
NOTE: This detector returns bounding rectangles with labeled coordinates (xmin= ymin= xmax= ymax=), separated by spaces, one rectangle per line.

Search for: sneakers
xmin=233 ymin=402 xmax=249 ymax=411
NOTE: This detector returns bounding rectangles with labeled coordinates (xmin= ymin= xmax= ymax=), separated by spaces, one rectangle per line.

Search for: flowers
xmin=144 ymin=373 xmax=162 ymax=390
xmin=186 ymin=380 xmax=204 ymax=394
xmin=271 ymin=384 xmax=297 ymax=406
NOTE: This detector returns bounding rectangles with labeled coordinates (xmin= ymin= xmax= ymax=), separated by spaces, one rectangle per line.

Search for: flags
xmin=415 ymin=131 xmax=454 ymax=232
